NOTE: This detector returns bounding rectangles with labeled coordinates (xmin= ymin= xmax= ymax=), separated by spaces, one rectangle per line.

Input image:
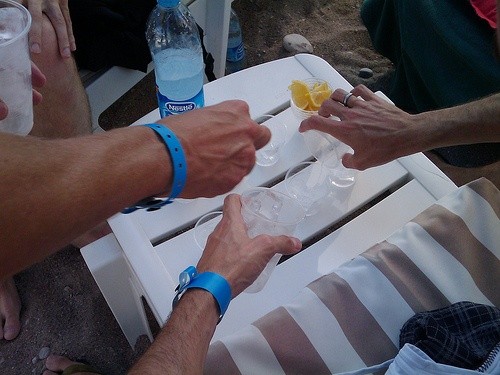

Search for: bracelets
xmin=172 ymin=265 xmax=233 ymax=324
xmin=120 ymin=122 xmax=186 ymax=214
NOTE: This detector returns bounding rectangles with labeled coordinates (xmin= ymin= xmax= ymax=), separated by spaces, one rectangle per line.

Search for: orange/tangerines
xmin=288 ymin=80 xmax=332 ymax=111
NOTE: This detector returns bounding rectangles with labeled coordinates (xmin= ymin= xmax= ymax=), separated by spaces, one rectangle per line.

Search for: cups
xmin=0 ymin=0 xmax=34 ymax=138
xmin=290 ymin=80 xmax=337 ymax=125
xmin=193 ymin=212 xmax=225 ymax=252
xmin=326 ymin=152 xmax=359 ymax=187
xmin=285 ymin=160 xmax=327 ymax=218
xmin=248 ymin=115 xmax=290 ymax=167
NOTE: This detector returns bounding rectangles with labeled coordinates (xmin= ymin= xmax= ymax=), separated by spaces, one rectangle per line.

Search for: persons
xmin=129 ymin=194 xmax=302 ymax=375
xmin=299 ymin=84 xmax=500 ymax=170
xmin=0 ymin=100 xmax=271 ymax=277
xmin=0 ymin=0 xmax=112 ymax=342
xmin=40 ymin=355 xmax=101 ymax=375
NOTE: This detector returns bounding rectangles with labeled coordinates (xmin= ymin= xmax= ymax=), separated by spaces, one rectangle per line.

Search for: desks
xmin=76 ymin=52 xmax=462 ymax=349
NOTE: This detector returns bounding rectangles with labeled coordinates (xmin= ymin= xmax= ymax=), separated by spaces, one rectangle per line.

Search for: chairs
xmin=83 ymin=0 xmax=237 ymax=140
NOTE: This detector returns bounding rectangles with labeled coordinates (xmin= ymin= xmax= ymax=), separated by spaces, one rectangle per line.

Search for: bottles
xmin=236 ymin=184 xmax=303 ymax=294
xmin=226 ymin=6 xmax=247 ymax=74
xmin=301 ymin=89 xmax=395 ymax=173
xmin=145 ymin=0 xmax=206 ymax=122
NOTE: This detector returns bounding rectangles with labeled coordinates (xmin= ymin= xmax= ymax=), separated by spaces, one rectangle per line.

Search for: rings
xmin=343 ymin=92 xmax=353 ymax=108
